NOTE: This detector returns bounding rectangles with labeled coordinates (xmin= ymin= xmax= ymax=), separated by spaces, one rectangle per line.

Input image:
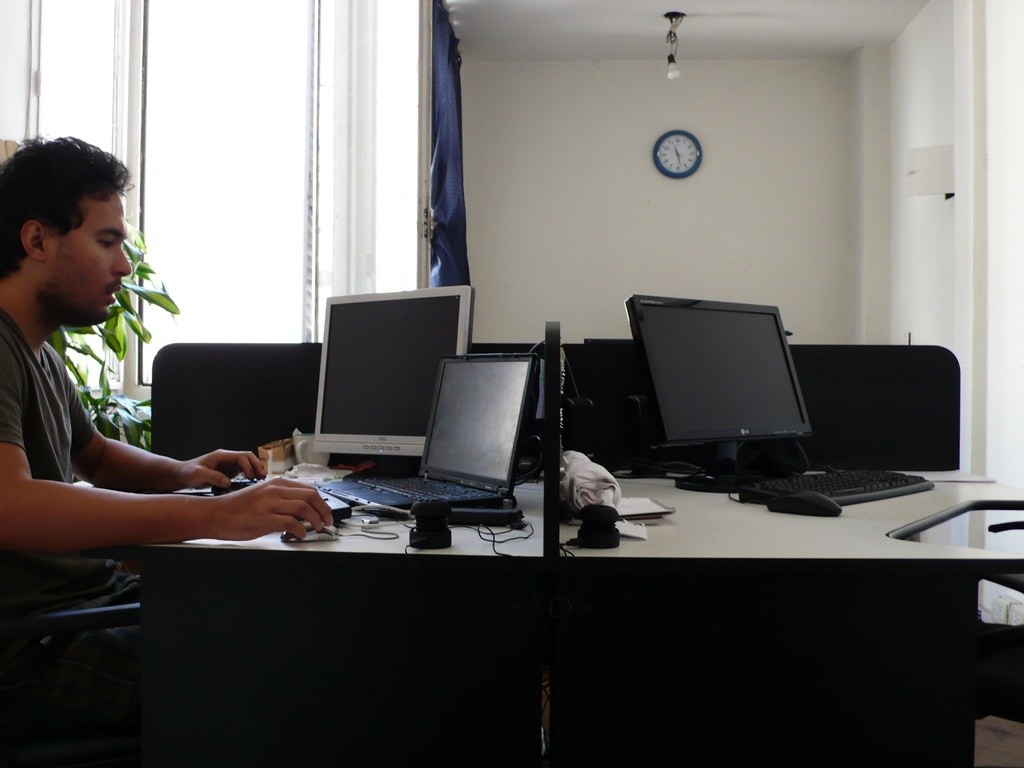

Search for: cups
xmin=294 ymin=433 xmax=330 ymax=467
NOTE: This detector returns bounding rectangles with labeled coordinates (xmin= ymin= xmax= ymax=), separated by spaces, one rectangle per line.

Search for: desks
xmin=83 ymin=322 xmax=1024 ymax=768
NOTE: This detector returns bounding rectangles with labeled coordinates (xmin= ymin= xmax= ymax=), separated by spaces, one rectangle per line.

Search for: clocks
xmin=653 ymin=130 xmax=702 ymax=179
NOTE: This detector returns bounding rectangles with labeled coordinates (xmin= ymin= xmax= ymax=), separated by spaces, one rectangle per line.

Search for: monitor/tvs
xmin=314 ymin=285 xmax=475 ymax=478
xmin=625 ymin=295 xmax=813 ymax=492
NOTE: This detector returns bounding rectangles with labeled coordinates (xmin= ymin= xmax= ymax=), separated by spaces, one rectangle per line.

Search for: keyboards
xmin=211 ymin=481 xmax=352 ymax=521
xmin=739 ymin=471 xmax=934 ymax=506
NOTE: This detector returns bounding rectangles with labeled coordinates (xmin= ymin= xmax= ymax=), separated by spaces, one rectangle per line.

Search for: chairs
xmin=975 ymin=522 xmax=1024 ymax=723
xmin=0 ymin=547 xmax=142 ymax=768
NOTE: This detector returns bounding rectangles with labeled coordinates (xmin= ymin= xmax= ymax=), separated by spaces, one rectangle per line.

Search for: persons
xmin=0 ymin=138 xmax=335 ymax=745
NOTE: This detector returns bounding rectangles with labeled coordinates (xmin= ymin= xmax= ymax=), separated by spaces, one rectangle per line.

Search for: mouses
xmin=281 ymin=521 xmax=339 ymax=541
xmin=768 ymin=490 xmax=842 ymax=517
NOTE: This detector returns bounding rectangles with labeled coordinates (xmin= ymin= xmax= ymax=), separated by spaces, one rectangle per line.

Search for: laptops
xmin=315 ymin=352 xmax=539 ymax=509
